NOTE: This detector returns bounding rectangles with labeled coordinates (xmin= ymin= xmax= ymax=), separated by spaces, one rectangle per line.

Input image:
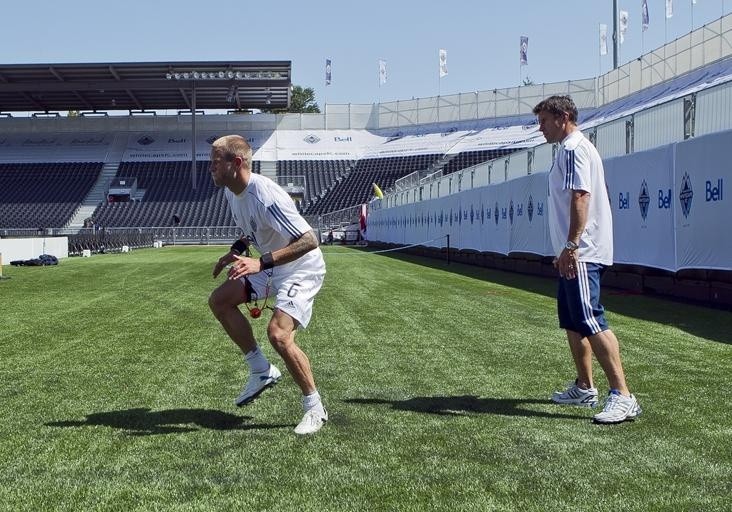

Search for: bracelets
xmin=230 ymin=239 xmax=248 ymax=256
xmin=259 ymin=251 xmax=274 ymax=271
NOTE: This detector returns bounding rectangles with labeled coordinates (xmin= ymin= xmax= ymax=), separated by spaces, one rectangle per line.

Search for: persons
xmin=327 ymin=232 xmax=333 ymax=245
xmin=208 ymin=134 xmax=329 ymax=435
xmin=532 ymin=94 xmax=642 ymax=423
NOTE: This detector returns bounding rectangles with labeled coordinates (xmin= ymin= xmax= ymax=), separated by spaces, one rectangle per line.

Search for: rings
xmin=568 ymin=264 xmax=574 ymax=269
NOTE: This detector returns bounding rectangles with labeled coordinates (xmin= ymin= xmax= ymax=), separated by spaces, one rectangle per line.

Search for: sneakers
xmin=592 ymin=389 xmax=643 ymax=424
xmin=230 ymin=363 xmax=282 ymax=409
xmin=550 ymin=379 xmax=599 ymax=408
xmin=291 ymin=406 xmax=329 ymax=437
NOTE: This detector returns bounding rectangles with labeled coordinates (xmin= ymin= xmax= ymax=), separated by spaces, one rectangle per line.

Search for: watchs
xmin=564 ymin=240 xmax=579 ymax=251
